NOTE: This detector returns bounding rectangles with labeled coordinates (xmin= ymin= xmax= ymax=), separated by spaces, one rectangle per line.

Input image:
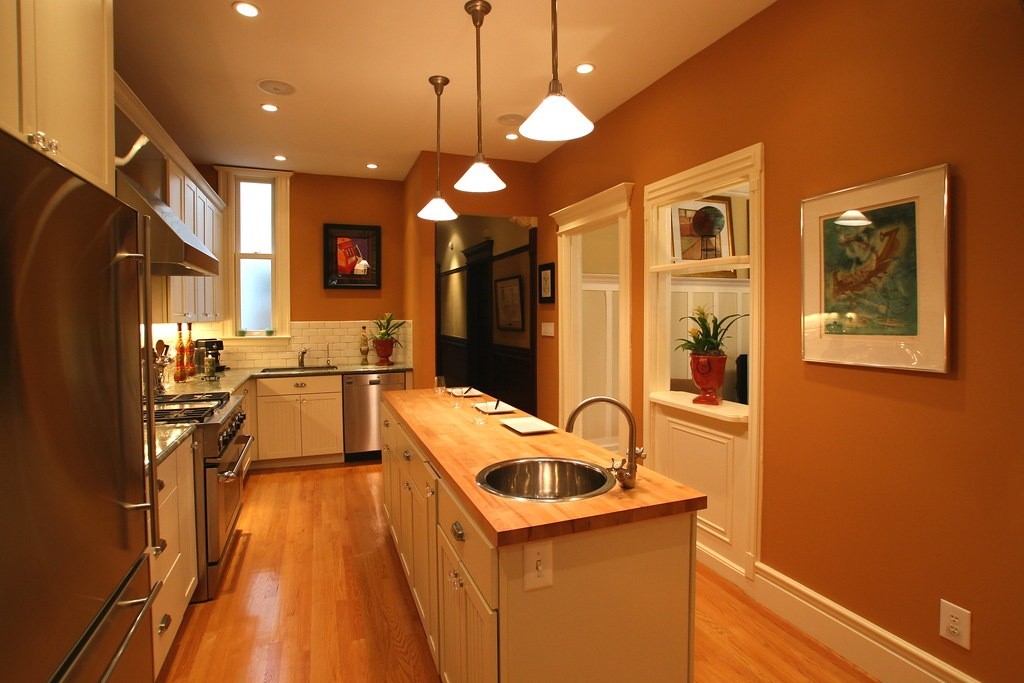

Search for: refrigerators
xmin=0 ymin=127 xmax=155 ymax=683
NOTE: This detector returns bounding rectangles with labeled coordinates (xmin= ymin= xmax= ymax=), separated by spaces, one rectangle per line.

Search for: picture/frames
xmin=671 ymin=196 xmax=738 ymax=278
xmin=495 ymin=274 xmax=525 ymax=332
xmin=323 ymin=223 xmax=381 ymax=289
xmin=799 ymin=163 xmax=953 ymax=375
xmin=538 ymin=262 xmax=555 ymax=304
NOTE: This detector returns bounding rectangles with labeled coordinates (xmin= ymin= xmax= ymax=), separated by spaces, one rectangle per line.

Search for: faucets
xmin=565 ymin=395 xmax=647 ymax=489
xmin=298 ymin=347 xmax=307 ymax=367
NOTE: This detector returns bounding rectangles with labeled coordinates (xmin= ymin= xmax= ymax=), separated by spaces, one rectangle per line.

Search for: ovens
xmin=203 ymin=434 xmax=255 ymax=598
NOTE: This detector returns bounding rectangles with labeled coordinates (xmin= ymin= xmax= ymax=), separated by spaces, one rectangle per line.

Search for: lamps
xmin=834 ymin=209 xmax=872 ymax=226
xmin=416 ymin=74 xmax=458 ymax=222
xmin=517 ymin=0 xmax=595 ymax=141
xmin=454 ymin=0 xmax=507 ymax=194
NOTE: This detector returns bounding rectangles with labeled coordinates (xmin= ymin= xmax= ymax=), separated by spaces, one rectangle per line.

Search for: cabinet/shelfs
xmin=648 ymin=255 xmax=750 ymax=423
xmin=144 ymin=435 xmax=200 ymax=681
xmin=140 ymin=158 xmax=224 ymax=323
xmin=232 ymin=380 xmax=252 ymax=479
xmin=256 ymin=375 xmax=344 ymax=460
xmin=0 ymin=0 xmax=116 ymax=197
xmin=378 ymin=399 xmax=499 ymax=683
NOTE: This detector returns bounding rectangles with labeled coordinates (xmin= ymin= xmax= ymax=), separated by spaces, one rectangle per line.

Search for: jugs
xmin=208 ymin=351 xmax=220 ymax=366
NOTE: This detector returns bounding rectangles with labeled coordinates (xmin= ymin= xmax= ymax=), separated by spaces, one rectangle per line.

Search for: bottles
xmin=174 ymin=323 xmax=187 ymax=383
xmin=185 ymin=323 xmax=195 ymax=377
xmin=359 ymin=325 xmax=369 ymax=365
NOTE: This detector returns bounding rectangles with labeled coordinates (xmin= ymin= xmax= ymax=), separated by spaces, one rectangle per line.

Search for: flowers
xmin=674 ymin=303 xmax=749 ymax=356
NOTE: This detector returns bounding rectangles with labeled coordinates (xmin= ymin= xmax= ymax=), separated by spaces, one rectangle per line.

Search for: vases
xmin=690 ymin=352 xmax=727 ymax=406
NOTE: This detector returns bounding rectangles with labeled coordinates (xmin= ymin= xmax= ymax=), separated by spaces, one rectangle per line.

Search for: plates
xmin=446 ymin=387 xmax=484 ymax=396
xmin=471 ymin=401 xmax=518 ymax=413
xmin=500 ymin=417 xmax=557 ymax=434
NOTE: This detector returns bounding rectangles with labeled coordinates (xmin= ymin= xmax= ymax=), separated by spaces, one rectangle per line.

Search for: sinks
xmin=260 ymin=365 xmax=339 ymax=372
xmin=474 ymin=456 xmax=616 ymax=503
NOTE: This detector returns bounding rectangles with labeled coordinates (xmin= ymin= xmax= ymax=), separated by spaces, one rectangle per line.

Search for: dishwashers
xmin=343 ymin=372 xmax=406 ymax=461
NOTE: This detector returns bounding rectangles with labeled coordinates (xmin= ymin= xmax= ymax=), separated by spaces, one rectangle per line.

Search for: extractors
xmin=115 ymin=169 xmax=220 ymax=277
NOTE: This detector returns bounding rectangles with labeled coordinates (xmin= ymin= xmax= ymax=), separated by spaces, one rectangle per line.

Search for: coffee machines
xmin=193 ymin=338 xmax=230 ymax=372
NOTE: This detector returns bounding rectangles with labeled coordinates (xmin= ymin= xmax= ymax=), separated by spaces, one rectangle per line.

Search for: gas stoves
xmin=141 ymin=347 xmax=247 ymax=458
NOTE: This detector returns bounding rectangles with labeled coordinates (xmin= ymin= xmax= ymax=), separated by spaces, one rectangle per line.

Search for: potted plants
xmin=366 ymin=311 xmax=407 ymax=366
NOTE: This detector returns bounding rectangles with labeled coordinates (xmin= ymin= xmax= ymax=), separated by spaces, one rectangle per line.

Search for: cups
xmin=204 ymin=357 xmax=215 ymax=375
xmin=265 ymin=328 xmax=274 ymax=336
xmin=473 ymin=401 xmax=488 ymax=424
xmin=237 ymin=328 xmax=247 ymax=337
xmin=450 ymin=387 xmax=464 ymax=408
xmin=433 ymin=377 xmax=446 ymax=394
